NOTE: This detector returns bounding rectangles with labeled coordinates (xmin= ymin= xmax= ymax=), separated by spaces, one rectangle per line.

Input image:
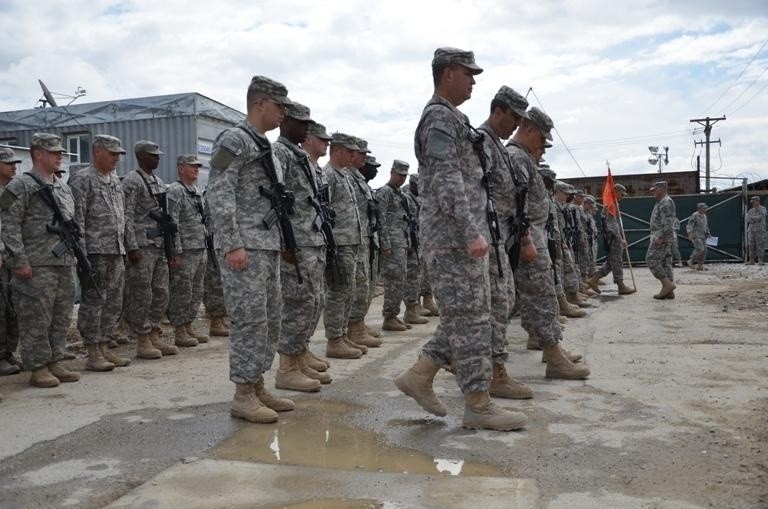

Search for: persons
xmin=685 ymin=202 xmax=712 ymax=271
xmin=744 ymin=196 xmax=767 ymax=265
xmin=644 ymin=181 xmax=675 ymax=300
xmin=391 ymin=45 xmax=528 ymax=430
xmin=669 ymin=217 xmax=683 ymax=268
xmin=585 ymin=183 xmax=635 ymax=295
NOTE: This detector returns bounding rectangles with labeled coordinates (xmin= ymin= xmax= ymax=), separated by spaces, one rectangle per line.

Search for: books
xmin=704 ymin=236 xmax=718 ymax=246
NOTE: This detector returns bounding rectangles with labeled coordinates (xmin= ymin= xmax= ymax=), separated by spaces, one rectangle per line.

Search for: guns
xmin=35 ymin=183 xmax=102 ymax=298
xmin=545 ymin=213 xmax=557 ymax=285
xmin=560 ymin=208 xmax=593 ymax=262
xmin=601 ymin=217 xmax=611 ymax=253
xmin=368 ymin=196 xmax=383 ymax=281
xmin=469 ymin=133 xmax=504 ymax=279
xmin=196 ymin=202 xmax=218 ymax=268
xmin=146 ymin=192 xmax=178 ymax=281
xmin=400 ymin=197 xmax=422 ymax=265
xmin=506 ymin=187 xmax=530 ymax=274
xmin=259 ymin=182 xmax=302 ymax=284
xmin=307 ymin=184 xmax=343 ymax=283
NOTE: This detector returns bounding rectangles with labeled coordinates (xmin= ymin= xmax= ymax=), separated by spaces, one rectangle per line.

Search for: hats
xmin=523 ymin=106 xmax=554 ymax=142
xmin=649 ymin=181 xmax=668 ymax=191
xmin=696 ymin=202 xmax=709 ymax=209
xmin=175 ymin=153 xmax=203 ymax=167
xmin=92 ymin=133 xmax=127 ymax=154
xmin=493 ymin=85 xmax=530 ymax=121
xmin=612 ymin=183 xmax=630 ymax=198
xmin=749 ymin=195 xmax=762 ymax=204
xmin=132 ymin=139 xmax=167 ymax=156
xmin=28 ymin=132 xmax=68 ymax=151
xmin=248 ymin=73 xmax=421 ymax=186
xmin=428 ymin=45 xmax=484 ymax=77
xmin=0 ymin=147 xmax=23 ymax=164
xmin=538 ymin=142 xmax=599 ymax=210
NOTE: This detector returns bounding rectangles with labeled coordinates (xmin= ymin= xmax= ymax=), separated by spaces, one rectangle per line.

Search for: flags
xmin=602 ymin=169 xmax=617 ymax=218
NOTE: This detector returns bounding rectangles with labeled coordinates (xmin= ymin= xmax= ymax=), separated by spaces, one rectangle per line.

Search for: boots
xmin=228 ymin=381 xmax=278 ymax=425
xmin=380 ymin=292 xmax=442 ymax=334
xmin=391 ymin=346 xmax=450 ymax=420
xmin=364 ymin=324 xmax=380 ymax=337
xmin=525 ymin=334 xmax=544 ymax=351
xmin=0 ymin=315 xmax=232 ymax=388
xmin=326 ymin=336 xmax=359 ymax=360
xmin=556 ymin=273 xmax=676 ymax=331
xmin=342 ymin=337 xmax=369 ymax=356
xmin=273 ymin=346 xmax=336 ymax=392
xmin=255 ymin=377 xmax=297 ymax=413
xmin=349 ymin=323 xmax=384 ymax=346
xmin=491 ymin=375 xmax=536 ymax=401
xmin=457 ymin=387 xmax=532 ymax=432
xmin=540 ymin=344 xmax=585 ymax=364
xmin=544 ymin=343 xmax=592 ymax=383
